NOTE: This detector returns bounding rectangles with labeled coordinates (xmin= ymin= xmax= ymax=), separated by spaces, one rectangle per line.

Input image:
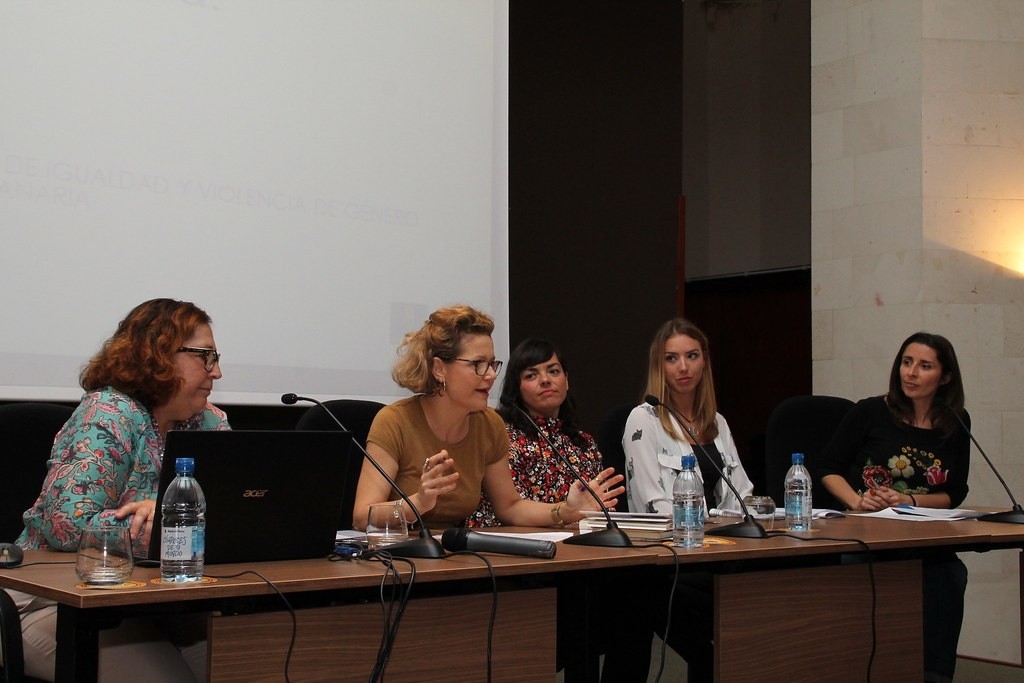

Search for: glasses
xmin=175 ymin=345 xmax=222 ymax=372
xmin=438 ymin=355 xmax=504 ymax=377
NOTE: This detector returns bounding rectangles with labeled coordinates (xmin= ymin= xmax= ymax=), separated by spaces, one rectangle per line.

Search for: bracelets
xmin=550 ymin=500 xmax=568 ymax=527
xmin=395 ymin=497 xmax=419 ymax=524
xmin=907 ymin=493 xmax=917 ymax=507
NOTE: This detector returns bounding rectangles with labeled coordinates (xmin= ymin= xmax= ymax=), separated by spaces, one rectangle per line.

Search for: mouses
xmin=0 ymin=542 xmax=24 ymax=567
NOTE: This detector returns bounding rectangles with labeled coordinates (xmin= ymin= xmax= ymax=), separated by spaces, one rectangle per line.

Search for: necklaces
xmin=673 ymin=408 xmax=699 ymax=437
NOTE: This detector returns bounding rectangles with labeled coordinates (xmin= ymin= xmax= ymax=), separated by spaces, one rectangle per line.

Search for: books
xmin=576 ymin=510 xmax=675 ymax=542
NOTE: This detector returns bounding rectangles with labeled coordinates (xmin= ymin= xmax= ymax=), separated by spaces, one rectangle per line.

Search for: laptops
xmin=97 ymin=430 xmax=353 ymax=565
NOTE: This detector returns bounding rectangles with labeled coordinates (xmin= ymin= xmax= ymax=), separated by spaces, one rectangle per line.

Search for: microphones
xmin=644 ymin=394 xmax=768 ymax=538
xmin=441 ymin=528 xmax=557 ymax=560
xmin=499 ymin=395 xmax=633 ymax=547
xmin=280 ymin=393 xmax=447 ymax=559
xmin=932 ymin=397 xmax=1024 ymax=524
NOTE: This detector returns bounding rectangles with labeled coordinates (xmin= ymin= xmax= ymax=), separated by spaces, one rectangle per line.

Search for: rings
xmin=423 ymin=458 xmax=431 ymax=470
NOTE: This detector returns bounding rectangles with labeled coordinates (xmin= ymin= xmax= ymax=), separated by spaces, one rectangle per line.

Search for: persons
xmin=353 ymin=304 xmax=628 ymax=526
xmin=0 ymin=298 xmax=236 ymax=682
xmin=818 ymin=333 xmax=972 ymax=683
xmin=622 ymin=316 xmax=754 ymax=517
xmin=464 ymin=335 xmax=656 ymax=683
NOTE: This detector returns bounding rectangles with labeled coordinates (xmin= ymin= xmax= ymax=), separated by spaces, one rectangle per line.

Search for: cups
xmin=366 ymin=504 xmax=408 ymax=549
xmin=741 ymin=496 xmax=775 ymax=530
xmin=75 ymin=525 xmax=134 ymax=585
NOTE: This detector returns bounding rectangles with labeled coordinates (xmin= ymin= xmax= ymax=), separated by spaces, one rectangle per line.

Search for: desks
xmin=0 ymin=507 xmax=1024 ymax=683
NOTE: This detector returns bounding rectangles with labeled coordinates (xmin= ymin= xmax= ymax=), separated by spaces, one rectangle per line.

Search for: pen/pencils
xmin=435 ymin=455 xmax=447 ymax=465
xmin=871 ymin=477 xmax=880 ymax=490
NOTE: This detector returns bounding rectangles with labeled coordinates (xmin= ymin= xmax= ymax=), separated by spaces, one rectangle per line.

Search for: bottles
xmin=673 ymin=455 xmax=704 ymax=548
xmin=160 ymin=458 xmax=208 ymax=582
xmin=784 ymin=453 xmax=813 ymax=530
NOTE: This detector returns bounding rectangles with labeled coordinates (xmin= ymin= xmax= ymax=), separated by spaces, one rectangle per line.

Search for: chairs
xmin=0 ymin=403 xmax=77 ymax=683
xmin=767 ymin=396 xmax=856 ymax=511
xmin=296 ymin=400 xmax=386 ymax=530
xmin=599 ymin=404 xmax=635 ymax=512
